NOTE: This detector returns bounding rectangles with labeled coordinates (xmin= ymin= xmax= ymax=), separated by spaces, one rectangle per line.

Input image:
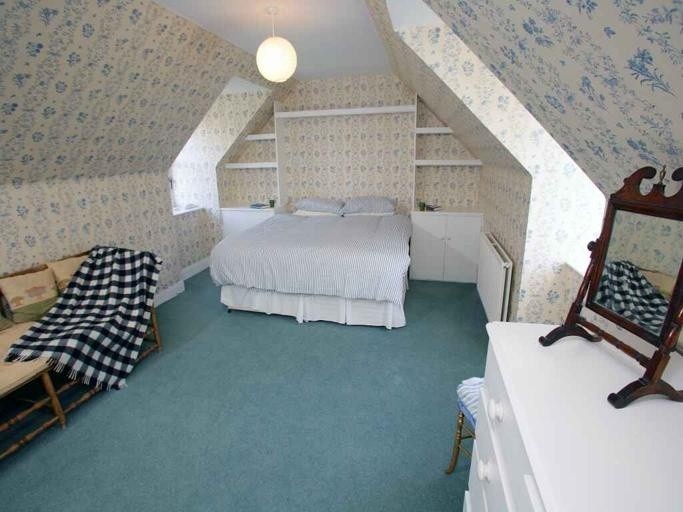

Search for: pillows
xmin=45 ymin=255 xmax=92 ymax=292
xmin=0 ymin=269 xmax=60 ymax=322
xmin=339 ymin=196 xmax=398 ymax=216
xmin=294 ymin=196 xmax=346 ymax=216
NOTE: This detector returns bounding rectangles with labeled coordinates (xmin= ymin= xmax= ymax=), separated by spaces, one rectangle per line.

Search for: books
xmin=426 ymin=203 xmax=443 ymax=212
xmin=250 ymin=202 xmax=271 ymax=209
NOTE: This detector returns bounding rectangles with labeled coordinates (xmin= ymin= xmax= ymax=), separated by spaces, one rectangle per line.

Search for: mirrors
xmin=536 ymin=161 xmax=683 ymax=413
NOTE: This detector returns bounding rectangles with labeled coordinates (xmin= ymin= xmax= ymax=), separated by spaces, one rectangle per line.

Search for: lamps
xmin=255 ymin=5 xmax=297 ymax=83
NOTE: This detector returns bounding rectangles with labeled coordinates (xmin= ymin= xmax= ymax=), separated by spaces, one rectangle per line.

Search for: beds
xmin=207 ymin=97 xmax=418 ymax=330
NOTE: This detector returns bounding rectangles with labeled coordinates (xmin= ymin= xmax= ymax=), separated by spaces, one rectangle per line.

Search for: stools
xmin=443 ymin=377 xmax=483 ymax=477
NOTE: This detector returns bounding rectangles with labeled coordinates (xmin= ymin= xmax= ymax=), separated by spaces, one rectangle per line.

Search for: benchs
xmin=0 ymin=246 xmax=161 ymax=466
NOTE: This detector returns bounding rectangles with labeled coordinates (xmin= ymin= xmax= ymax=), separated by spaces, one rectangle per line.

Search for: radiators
xmin=475 ymin=231 xmax=513 ymax=322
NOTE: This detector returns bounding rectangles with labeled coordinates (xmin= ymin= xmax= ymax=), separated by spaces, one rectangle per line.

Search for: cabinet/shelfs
xmin=410 ymin=207 xmax=484 ymax=285
xmin=222 ymin=133 xmax=277 ymax=170
xmin=415 ymin=126 xmax=481 ymax=168
xmin=216 ymin=203 xmax=272 ymax=241
xmin=461 ymin=321 xmax=682 ymax=512
